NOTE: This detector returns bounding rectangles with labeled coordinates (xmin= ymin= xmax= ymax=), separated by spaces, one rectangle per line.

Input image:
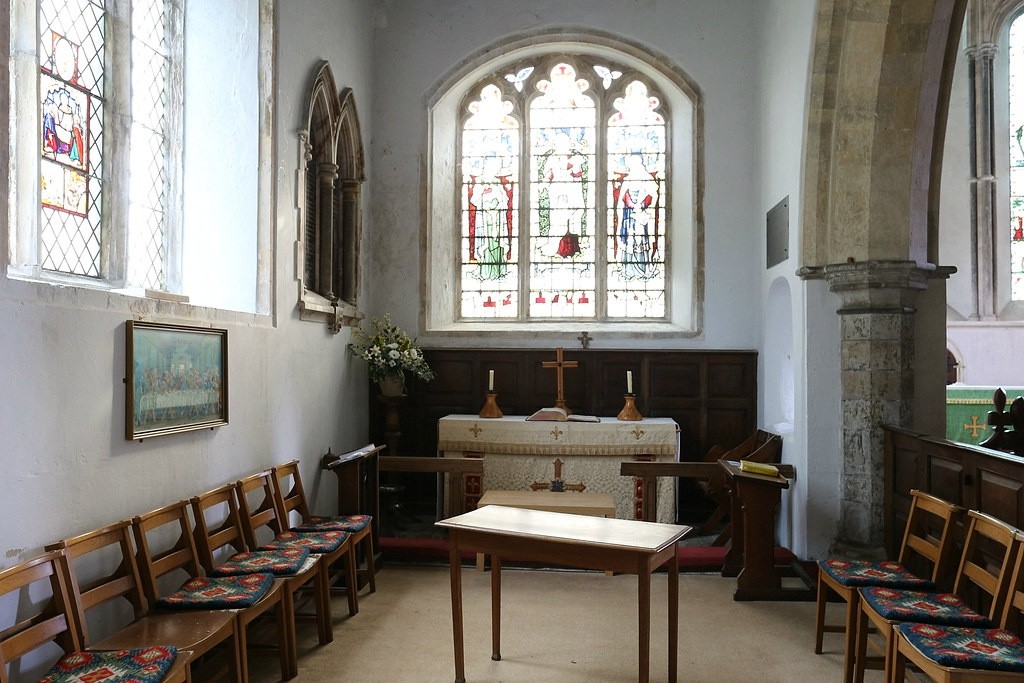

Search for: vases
xmin=378 ymin=368 xmax=405 ymax=397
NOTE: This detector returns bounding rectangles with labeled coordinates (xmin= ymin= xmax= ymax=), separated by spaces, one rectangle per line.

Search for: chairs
xmin=854 ymin=510 xmax=1018 ymax=683
xmin=0 ymin=461 xmax=377 ymax=683
xmin=890 ymin=532 xmax=1024 ymax=683
xmin=815 ymin=490 xmax=964 ymax=683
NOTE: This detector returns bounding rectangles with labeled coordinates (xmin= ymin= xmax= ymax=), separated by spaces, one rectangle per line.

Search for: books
xmin=525 ymin=408 xmax=601 ymax=423
xmin=739 ymin=460 xmax=778 ymax=478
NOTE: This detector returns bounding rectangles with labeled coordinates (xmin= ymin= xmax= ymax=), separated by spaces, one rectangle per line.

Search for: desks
xmin=438 ymin=414 xmax=680 ymax=527
xmin=476 ymin=490 xmax=615 ymax=577
xmin=434 ymin=505 xmax=692 ymax=683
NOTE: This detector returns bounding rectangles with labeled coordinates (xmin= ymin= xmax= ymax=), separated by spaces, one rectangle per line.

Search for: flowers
xmin=345 ymin=314 xmax=434 ymax=384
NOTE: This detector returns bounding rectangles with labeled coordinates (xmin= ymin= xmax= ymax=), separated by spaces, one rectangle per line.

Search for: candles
xmin=627 ymin=371 xmax=633 ymax=396
xmin=489 ymin=369 xmax=494 ymax=392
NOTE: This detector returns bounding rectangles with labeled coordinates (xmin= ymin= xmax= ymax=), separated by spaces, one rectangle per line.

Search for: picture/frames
xmin=126 ymin=319 xmax=230 ymax=440
